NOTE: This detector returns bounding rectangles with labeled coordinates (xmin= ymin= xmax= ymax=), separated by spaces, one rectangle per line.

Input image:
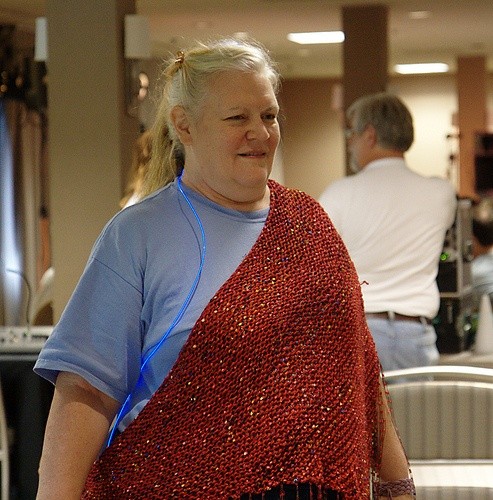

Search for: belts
xmin=366 ymin=312 xmax=431 ymax=325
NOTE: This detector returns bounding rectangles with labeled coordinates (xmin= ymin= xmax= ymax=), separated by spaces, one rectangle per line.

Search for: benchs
xmin=384 ymin=366 xmax=493 ymax=500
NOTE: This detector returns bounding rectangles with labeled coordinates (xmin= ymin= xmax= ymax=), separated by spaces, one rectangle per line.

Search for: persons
xmin=32 ymin=39 xmax=417 ymax=500
xmin=470 ymin=202 xmax=493 ymax=301
xmin=312 ymin=94 xmax=458 ymax=385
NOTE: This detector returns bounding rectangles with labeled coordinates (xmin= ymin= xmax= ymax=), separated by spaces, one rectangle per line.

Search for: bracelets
xmin=376 ymin=478 xmax=416 ymax=497
